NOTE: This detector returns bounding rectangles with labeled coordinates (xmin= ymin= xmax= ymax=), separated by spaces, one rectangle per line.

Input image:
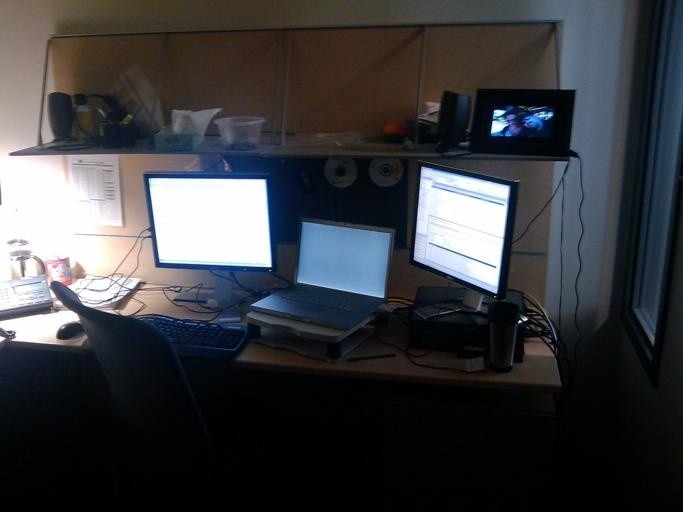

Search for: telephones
xmin=0 ymin=273 xmax=54 ymax=320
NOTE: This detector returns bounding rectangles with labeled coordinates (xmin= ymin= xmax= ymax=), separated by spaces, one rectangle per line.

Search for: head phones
xmin=75 ymin=91 xmax=140 ymax=148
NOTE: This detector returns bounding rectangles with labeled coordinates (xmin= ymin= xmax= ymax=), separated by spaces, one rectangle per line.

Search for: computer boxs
xmin=408 ymin=286 xmax=526 ymax=357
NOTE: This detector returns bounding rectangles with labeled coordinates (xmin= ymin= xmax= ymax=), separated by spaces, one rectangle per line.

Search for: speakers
xmin=47 ymin=92 xmax=71 ymax=142
xmin=435 ymin=89 xmax=472 ymax=153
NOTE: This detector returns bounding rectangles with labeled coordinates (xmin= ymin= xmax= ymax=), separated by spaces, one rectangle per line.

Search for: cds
xmin=323 ymin=158 xmax=358 ymax=189
xmin=368 ymin=158 xmax=404 ymax=188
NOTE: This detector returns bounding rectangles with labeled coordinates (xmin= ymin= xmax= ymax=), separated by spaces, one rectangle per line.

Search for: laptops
xmin=470 ymin=88 xmax=575 ymax=157
xmin=249 ymin=216 xmax=397 ymax=328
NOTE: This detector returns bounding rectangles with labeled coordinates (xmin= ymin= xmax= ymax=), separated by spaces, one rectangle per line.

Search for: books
xmin=54 ymin=274 xmax=141 ymax=311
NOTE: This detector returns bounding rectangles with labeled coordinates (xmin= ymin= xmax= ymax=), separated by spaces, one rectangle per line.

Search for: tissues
xmin=155 ymin=108 xmax=222 ymax=152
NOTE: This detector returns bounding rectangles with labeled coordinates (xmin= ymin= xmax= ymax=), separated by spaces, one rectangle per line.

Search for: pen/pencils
xmin=346 ymin=353 xmax=397 ymax=361
xmin=172 ymin=298 xmax=208 ymax=304
xmin=96 ymin=102 xmax=142 ymax=124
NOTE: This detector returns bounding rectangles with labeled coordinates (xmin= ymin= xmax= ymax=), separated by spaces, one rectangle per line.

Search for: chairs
xmin=48 ymin=282 xmax=258 ymax=495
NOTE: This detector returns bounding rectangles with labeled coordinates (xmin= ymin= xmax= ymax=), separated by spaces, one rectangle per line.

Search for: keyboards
xmin=129 ymin=314 xmax=250 ymax=353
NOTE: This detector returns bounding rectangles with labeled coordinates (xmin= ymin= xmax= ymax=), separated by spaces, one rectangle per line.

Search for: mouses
xmin=55 ymin=320 xmax=83 ymax=339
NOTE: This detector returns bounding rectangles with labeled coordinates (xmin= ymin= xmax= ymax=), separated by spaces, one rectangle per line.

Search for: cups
xmin=486 ymin=301 xmax=521 ymax=373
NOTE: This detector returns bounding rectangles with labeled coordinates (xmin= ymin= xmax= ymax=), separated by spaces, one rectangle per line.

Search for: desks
xmin=0 ymin=276 xmax=562 ymax=399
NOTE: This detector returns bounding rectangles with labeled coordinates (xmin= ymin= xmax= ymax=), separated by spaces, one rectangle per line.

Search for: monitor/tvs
xmin=408 ymin=159 xmax=530 ymax=328
xmin=143 ymin=172 xmax=279 ymax=323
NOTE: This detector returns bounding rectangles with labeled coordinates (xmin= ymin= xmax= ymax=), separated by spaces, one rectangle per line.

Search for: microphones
xmin=74 ymin=93 xmax=104 ymax=106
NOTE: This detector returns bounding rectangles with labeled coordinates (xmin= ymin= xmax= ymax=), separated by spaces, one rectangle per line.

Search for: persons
xmin=490 ymin=109 xmax=535 ymax=137
xmin=518 ymin=112 xmax=554 ymax=136
